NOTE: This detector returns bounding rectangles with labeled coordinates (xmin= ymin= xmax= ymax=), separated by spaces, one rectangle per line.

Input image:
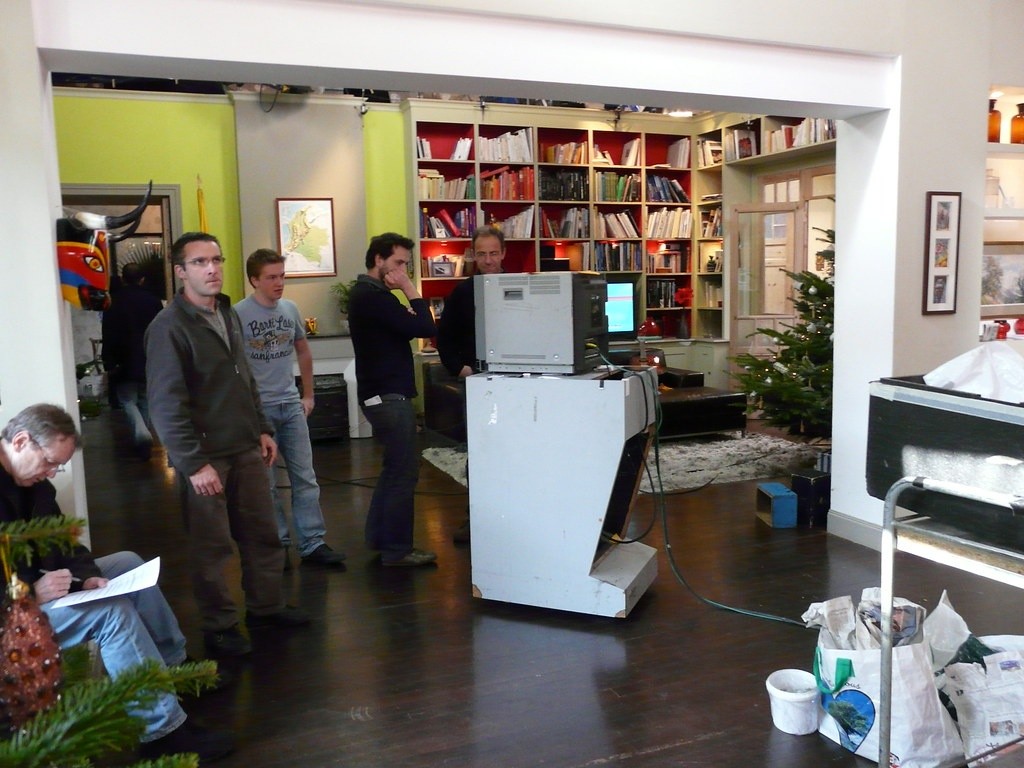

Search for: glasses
xmin=28 ymin=434 xmax=65 ymax=473
xmin=179 ymin=255 xmax=225 ymax=266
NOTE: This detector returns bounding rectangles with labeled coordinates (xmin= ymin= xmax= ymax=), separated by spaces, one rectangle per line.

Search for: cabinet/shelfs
xmin=399 ymin=98 xmax=836 ymax=417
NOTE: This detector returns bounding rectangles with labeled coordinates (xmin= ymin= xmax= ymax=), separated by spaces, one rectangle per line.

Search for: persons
xmin=142 ymin=231 xmax=323 ymax=656
xmin=0 ymin=405 xmax=238 ymax=762
xmin=101 ymin=262 xmax=176 ymax=484
xmin=348 ymin=234 xmax=438 ymax=565
xmin=232 ymin=248 xmax=346 ymax=567
xmin=435 ymin=225 xmax=506 ymax=547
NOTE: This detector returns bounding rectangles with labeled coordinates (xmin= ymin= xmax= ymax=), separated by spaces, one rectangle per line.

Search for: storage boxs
xmin=791 ymin=469 xmax=831 ymax=530
xmin=754 ymin=482 xmax=797 ymax=529
xmin=865 ymin=375 xmax=1024 ymax=549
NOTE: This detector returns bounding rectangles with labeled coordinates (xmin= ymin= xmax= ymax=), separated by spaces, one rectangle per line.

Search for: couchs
xmin=423 ymin=348 xmax=705 ymax=444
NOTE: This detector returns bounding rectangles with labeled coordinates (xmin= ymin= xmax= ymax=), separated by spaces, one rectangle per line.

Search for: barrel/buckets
xmin=766 ymin=667 xmax=822 ymax=735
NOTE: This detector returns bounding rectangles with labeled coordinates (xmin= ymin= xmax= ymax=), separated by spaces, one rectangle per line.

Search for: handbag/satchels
xmin=813 ymin=631 xmax=965 ymax=768
xmin=924 ymin=588 xmax=998 ymax=725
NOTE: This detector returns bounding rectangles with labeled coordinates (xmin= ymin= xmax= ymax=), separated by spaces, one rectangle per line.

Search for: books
xmin=594 ymin=243 xmax=641 ymax=270
xmin=650 ymin=138 xmax=690 ymax=168
xmin=726 ymin=118 xmax=836 ymax=162
xmin=539 ymin=141 xmax=587 ymax=164
xmin=647 ymin=175 xmax=691 ymax=202
xmin=538 ymin=169 xmax=589 ymax=201
xmin=540 ymin=242 xmax=589 ymax=270
xmin=646 ymin=208 xmax=691 ymax=307
xmin=593 ymin=206 xmax=641 ymax=238
xmin=540 ymin=207 xmax=589 ymax=238
xmin=697 ymin=139 xmax=721 ymax=308
xmin=594 ymin=138 xmax=640 ymax=166
xmin=593 ymin=170 xmax=641 ymax=202
xmin=416 ymin=128 xmax=535 ymax=278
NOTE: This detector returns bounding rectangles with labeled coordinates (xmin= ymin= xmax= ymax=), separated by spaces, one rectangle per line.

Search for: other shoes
xmin=301 ymin=543 xmax=347 ymax=562
xmin=177 ymin=655 xmax=237 ymax=717
xmin=452 ymin=516 xmax=470 ymax=539
xmin=245 ymin=604 xmax=309 ymax=626
xmin=202 ymin=624 xmax=254 ymax=653
xmin=142 ymin=720 xmax=231 ymax=762
xmin=380 ymin=549 xmax=436 ymax=566
xmin=280 ymin=535 xmax=291 ymax=571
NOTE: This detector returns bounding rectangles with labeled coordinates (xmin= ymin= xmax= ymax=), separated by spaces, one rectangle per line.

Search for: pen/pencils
xmin=39 ymin=568 xmax=81 ymax=582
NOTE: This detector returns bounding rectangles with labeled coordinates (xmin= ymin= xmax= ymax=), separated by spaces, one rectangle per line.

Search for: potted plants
xmin=329 ymin=279 xmax=356 ymax=334
xmin=721 ymin=194 xmax=835 ymax=472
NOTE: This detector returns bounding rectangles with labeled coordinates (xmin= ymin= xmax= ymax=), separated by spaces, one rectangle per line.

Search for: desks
xmin=654 ymin=387 xmax=747 ymax=442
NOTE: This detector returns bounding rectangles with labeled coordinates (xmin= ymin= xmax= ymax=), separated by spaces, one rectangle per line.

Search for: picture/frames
xmin=922 ymin=191 xmax=962 ymax=315
xmin=981 ymin=242 xmax=1024 ymax=320
xmin=276 ymin=198 xmax=337 ymax=278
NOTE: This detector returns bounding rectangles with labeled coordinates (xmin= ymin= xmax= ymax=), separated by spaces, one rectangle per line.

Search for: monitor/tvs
xmin=604 ymin=281 xmax=638 ymax=342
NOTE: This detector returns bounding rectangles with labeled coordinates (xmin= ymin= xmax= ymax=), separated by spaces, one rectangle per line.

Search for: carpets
xmin=421 ymin=431 xmax=818 ymax=494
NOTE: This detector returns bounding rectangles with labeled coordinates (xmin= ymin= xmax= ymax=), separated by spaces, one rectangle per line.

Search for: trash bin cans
xmin=765 ymin=669 xmax=821 ymax=735
